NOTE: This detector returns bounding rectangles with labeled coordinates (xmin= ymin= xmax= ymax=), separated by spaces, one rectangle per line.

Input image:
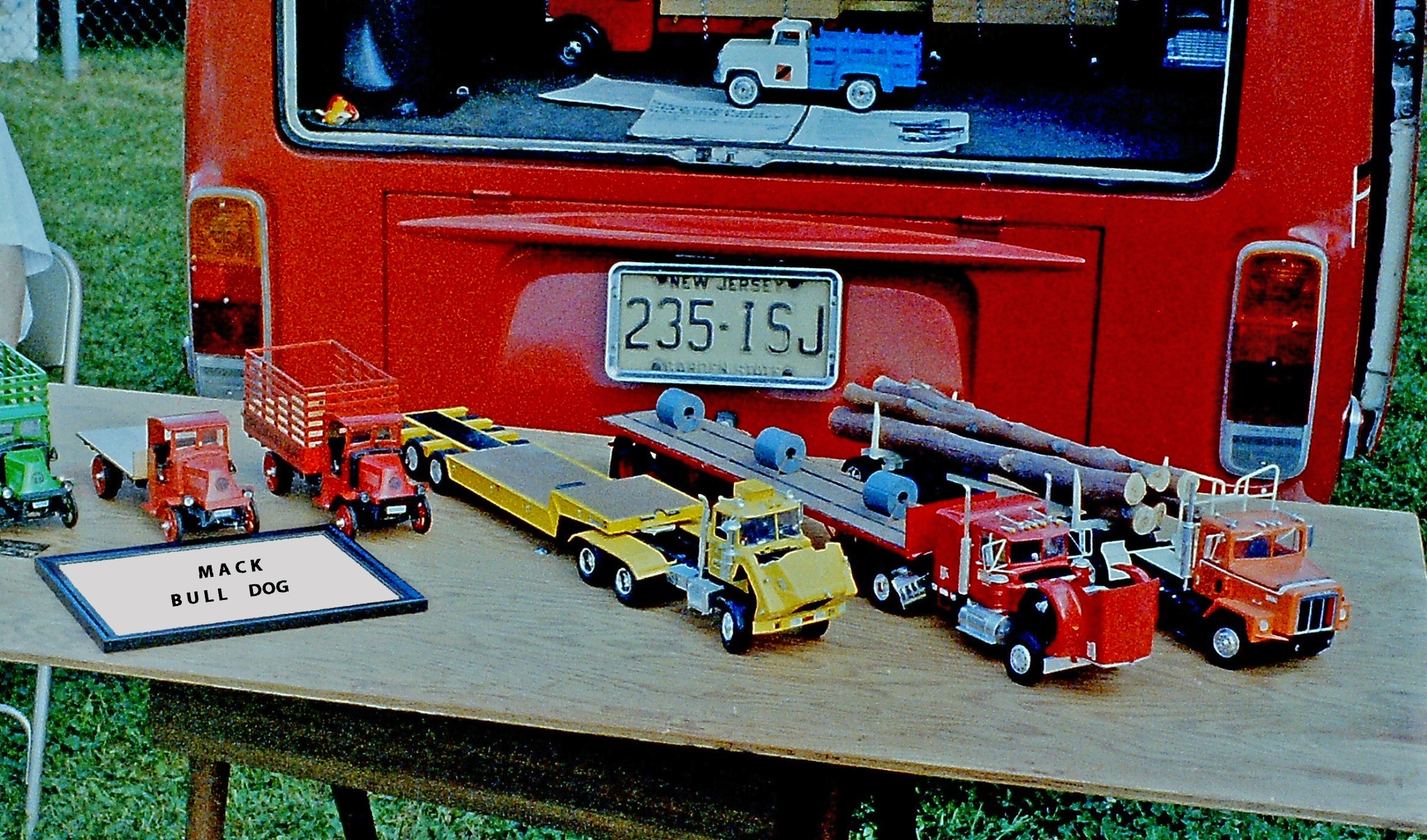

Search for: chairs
xmin=0 ymin=237 xmax=83 ymax=840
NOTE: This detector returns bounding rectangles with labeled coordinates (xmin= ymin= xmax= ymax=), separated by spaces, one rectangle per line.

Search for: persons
xmin=1 ymin=112 xmax=54 ymax=354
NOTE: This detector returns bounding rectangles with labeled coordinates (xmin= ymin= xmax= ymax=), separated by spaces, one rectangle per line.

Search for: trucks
xmin=0 ymin=339 xmax=79 ymax=530
xmin=77 ymin=409 xmax=260 ymax=544
xmin=242 ymin=338 xmax=432 ymax=539
xmin=712 ymin=16 xmax=928 ymax=114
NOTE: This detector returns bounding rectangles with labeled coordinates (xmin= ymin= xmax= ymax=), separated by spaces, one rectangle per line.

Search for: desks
xmin=0 ymin=385 xmax=1427 ymax=840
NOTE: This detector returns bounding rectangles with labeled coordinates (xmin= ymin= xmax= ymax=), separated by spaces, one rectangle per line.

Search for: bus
xmin=179 ymin=0 xmax=1426 ymax=506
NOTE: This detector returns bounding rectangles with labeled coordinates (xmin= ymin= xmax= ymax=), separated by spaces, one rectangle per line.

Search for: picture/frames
xmin=32 ymin=518 xmax=427 ymax=654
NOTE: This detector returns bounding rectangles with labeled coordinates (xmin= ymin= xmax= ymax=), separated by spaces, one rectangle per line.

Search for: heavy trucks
xmin=601 ymin=387 xmax=1161 ymax=686
xmin=400 ymin=406 xmax=858 ymax=656
xmin=842 ymin=418 xmax=1350 ymax=671
